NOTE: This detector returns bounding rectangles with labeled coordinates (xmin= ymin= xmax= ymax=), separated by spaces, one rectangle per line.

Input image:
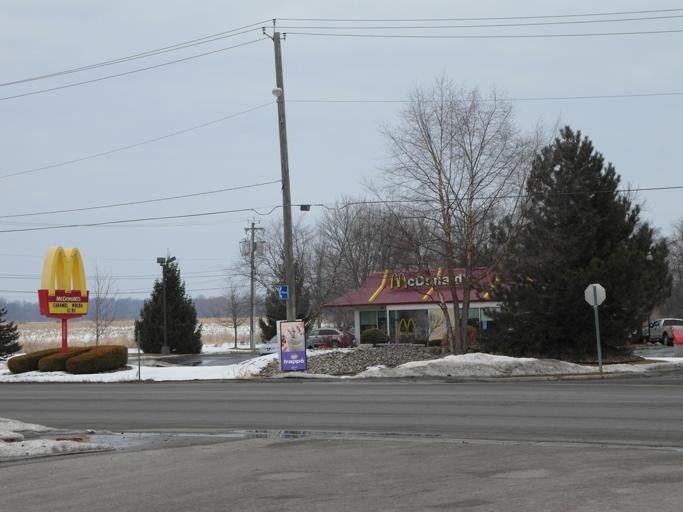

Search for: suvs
xmin=642 ymin=318 xmax=682 ymax=347
xmin=308 ymin=327 xmax=354 ymax=349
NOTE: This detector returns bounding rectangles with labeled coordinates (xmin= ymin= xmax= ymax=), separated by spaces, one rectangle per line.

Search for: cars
xmin=254 ymin=333 xmax=314 ymax=356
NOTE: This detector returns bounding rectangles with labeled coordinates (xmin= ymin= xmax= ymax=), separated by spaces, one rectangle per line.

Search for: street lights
xmin=269 ymin=87 xmax=298 ymax=320
xmin=155 ymin=256 xmax=177 ymax=355
